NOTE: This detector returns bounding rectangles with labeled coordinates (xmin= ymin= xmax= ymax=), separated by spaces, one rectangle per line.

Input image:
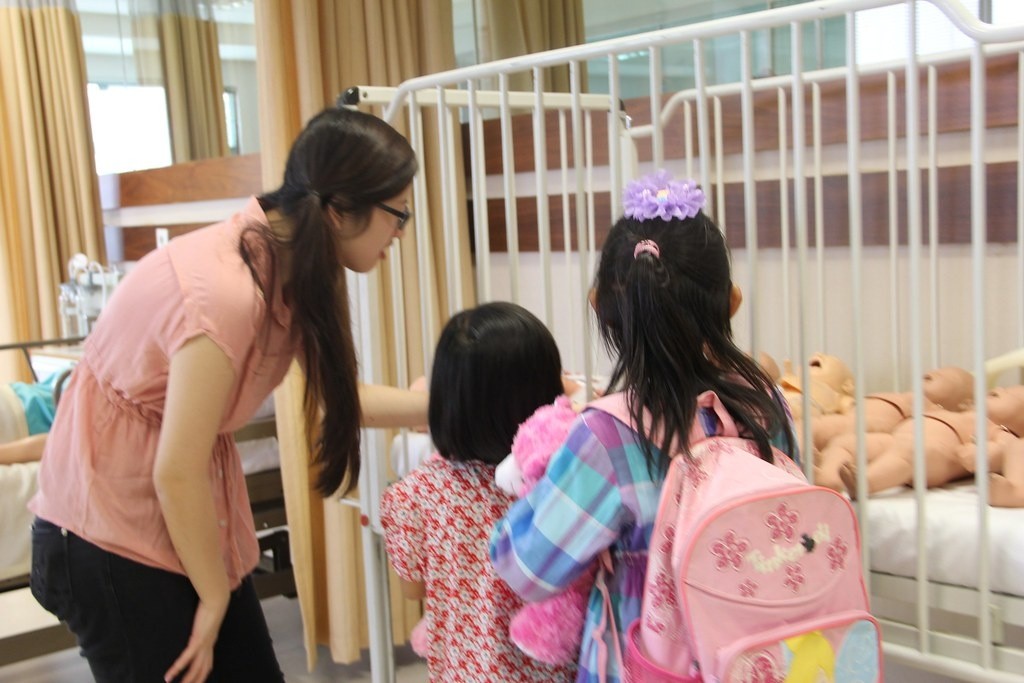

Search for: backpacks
xmin=576 ymin=391 xmax=883 ymax=683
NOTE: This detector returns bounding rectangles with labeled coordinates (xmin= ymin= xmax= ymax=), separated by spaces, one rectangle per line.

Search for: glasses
xmin=374 ymin=199 xmax=411 ymax=228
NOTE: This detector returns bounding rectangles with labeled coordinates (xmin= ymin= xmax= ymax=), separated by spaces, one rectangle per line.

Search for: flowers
xmin=621 ymin=169 xmax=705 ymax=221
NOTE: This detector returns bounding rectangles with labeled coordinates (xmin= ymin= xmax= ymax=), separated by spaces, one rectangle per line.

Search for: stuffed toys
xmin=494 ymin=395 xmax=600 ymax=666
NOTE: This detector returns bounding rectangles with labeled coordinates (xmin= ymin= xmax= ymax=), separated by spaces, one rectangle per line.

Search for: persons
xmin=28 ymin=107 xmax=429 ymax=683
xmin=723 ymin=352 xmax=1023 ymax=508
xmin=489 ymin=169 xmax=799 ymax=683
xmin=378 ymin=301 xmax=598 ymax=683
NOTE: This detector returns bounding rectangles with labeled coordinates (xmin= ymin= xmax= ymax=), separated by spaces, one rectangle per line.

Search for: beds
xmin=1 ymin=191 xmax=290 ymax=666
xmin=337 ymin=1 xmax=1024 ymax=683
